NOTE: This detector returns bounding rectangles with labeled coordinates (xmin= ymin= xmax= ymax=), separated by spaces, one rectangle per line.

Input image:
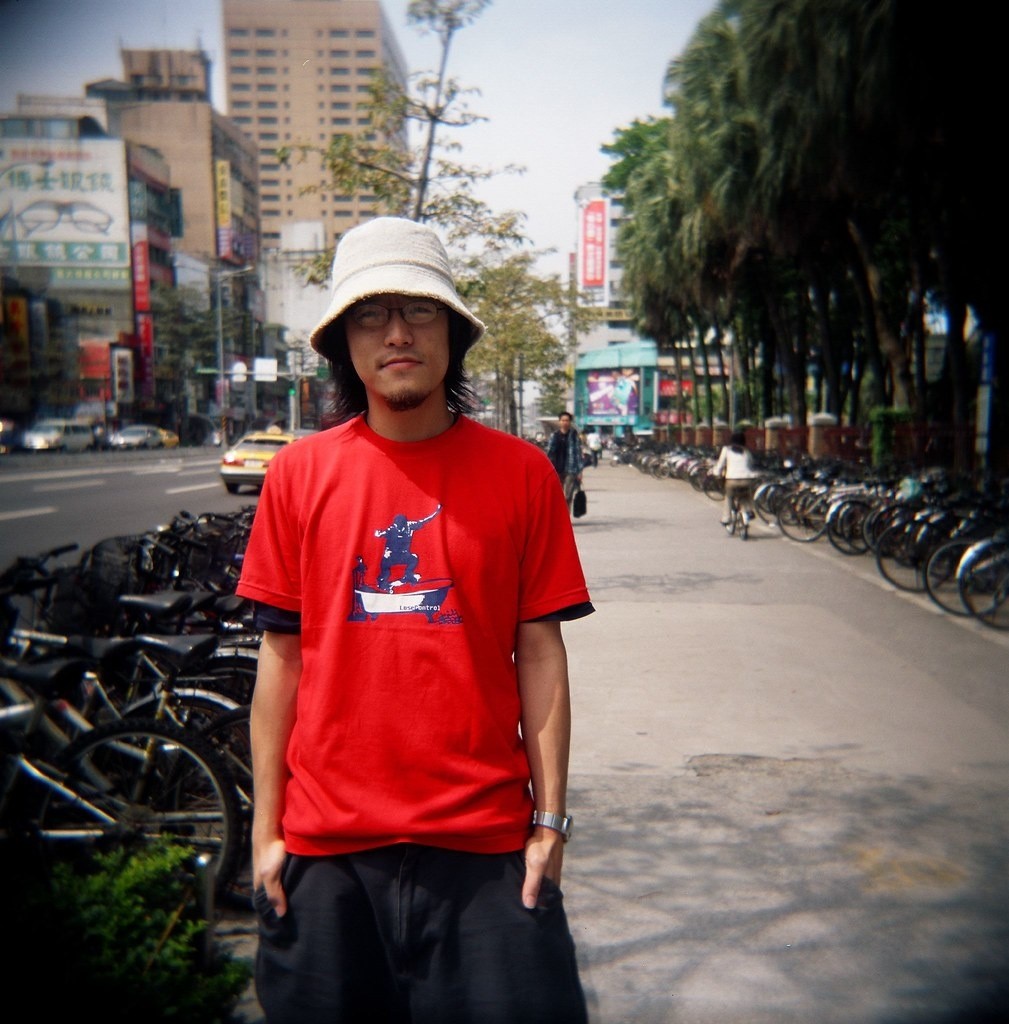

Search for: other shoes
xmin=721 ymin=520 xmax=729 ymax=525
xmin=748 ymin=511 xmax=754 ymax=519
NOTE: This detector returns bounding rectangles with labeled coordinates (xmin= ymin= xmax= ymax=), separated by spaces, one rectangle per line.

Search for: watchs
xmin=533 ymin=810 xmax=574 ymax=842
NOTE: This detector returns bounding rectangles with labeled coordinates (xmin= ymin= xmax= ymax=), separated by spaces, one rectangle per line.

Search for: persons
xmin=578 ymin=427 xmax=601 ymax=468
xmin=711 ymin=433 xmax=755 ymax=526
xmin=235 ymin=217 xmax=596 ymax=1024
xmin=547 ymin=412 xmax=581 ymax=515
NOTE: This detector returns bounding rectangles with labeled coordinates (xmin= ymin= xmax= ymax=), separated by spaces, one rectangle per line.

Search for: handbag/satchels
xmin=574 ymin=491 xmax=588 ymax=517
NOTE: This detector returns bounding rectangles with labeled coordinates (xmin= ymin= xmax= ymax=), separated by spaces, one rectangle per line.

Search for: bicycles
xmin=610 ymin=438 xmax=1009 ymax=632
xmin=717 ymin=476 xmax=754 ymax=541
xmin=0 ymin=504 xmax=264 ymax=911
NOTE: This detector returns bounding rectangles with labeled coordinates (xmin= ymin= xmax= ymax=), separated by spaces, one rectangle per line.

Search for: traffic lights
xmin=288 ymin=381 xmax=296 ymax=395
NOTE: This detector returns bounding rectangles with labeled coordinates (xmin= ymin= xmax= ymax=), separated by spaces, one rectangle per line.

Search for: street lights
xmin=218 ymin=264 xmax=253 ymax=433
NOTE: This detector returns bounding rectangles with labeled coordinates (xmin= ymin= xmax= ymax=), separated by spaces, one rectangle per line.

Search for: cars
xmin=107 ymin=424 xmax=179 ymax=450
xmin=220 ymin=432 xmax=296 ymax=494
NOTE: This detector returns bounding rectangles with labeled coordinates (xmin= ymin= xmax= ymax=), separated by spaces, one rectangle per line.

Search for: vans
xmin=23 ymin=417 xmax=95 ymax=453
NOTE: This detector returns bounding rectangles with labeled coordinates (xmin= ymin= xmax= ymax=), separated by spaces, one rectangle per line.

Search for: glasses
xmin=349 ymin=301 xmax=443 ymax=328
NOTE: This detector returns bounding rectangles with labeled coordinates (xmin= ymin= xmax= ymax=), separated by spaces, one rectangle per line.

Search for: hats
xmin=308 ymin=217 xmax=487 ymax=361
